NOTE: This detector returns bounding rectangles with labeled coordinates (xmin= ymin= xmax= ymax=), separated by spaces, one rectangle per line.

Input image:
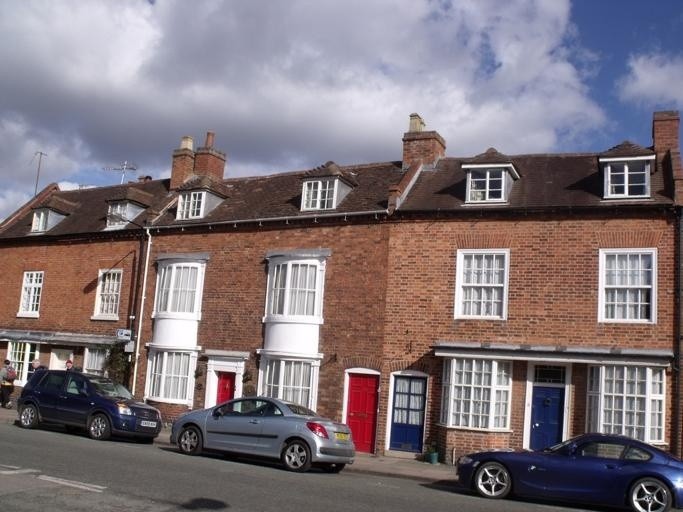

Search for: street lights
xmin=107 ymin=211 xmax=151 ymax=395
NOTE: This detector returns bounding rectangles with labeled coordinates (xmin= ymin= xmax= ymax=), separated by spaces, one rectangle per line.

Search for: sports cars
xmin=455 ymin=432 xmax=683 ymax=512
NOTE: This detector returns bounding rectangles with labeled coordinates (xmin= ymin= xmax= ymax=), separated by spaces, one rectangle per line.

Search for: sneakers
xmin=6 ymin=403 xmax=13 ymax=409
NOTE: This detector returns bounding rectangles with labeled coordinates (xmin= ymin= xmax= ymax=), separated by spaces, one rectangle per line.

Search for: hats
xmin=66 ymin=360 xmax=72 ymax=364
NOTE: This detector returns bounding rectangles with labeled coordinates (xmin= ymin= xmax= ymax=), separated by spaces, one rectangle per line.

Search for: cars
xmin=170 ymin=396 xmax=356 ymax=473
xmin=17 ymin=368 xmax=163 ymax=440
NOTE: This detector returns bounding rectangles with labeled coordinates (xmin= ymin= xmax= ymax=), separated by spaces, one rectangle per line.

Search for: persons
xmin=31 ymin=360 xmax=47 ymax=373
xmin=0 ymin=360 xmax=15 ymax=409
xmin=66 ymin=360 xmax=80 ymax=371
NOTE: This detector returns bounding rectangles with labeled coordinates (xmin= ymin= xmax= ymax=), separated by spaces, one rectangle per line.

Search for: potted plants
xmin=426 ymin=447 xmax=438 ymax=464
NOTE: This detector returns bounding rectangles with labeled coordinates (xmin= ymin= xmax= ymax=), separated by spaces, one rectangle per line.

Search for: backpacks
xmin=2 ymin=366 xmax=17 ymax=383
